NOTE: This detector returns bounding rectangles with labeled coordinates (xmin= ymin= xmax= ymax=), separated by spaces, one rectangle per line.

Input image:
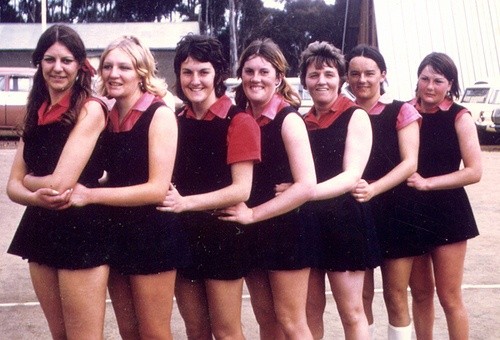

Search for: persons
xmin=213 ymin=38 xmax=317 ymax=340
xmin=155 ymin=35 xmax=261 ymax=340
xmin=6 ymin=24 xmax=110 ymax=340
xmin=69 ymin=36 xmax=191 ymax=340
xmin=405 ymin=53 xmax=482 ymax=340
xmin=274 ymin=40 xmax=384 ymax=340
xmin=346 ymin=43 xmax=438 ymax=340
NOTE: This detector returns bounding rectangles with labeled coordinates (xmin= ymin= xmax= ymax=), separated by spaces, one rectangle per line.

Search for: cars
xmin=224 ymin=77 xmax=356 ymax=114
xmin=460 ymin=82 xmax=500 ymax=144
xmin=0 ymin=67 xmax=39 ymax=137
